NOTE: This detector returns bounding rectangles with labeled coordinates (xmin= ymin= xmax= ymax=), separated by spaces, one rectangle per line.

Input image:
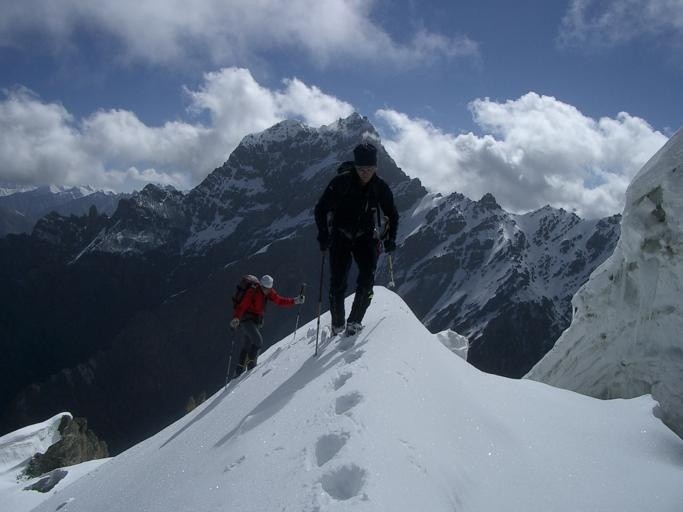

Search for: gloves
xmin=231 ymin=318 xmax=239 ymax=328
xmin=294 ymin=295 xmax=306 ymax=304
xmin=317 ymin=232 xmax=331 ymax=250
xmin=384 ymin=240 xmax=395 ymax=253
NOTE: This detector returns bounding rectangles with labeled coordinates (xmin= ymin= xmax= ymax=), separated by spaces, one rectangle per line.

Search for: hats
xmin=261 ymin=275 xmax=275 ymax=289
xmin=353 ymin=143 xmax=377 ymax=167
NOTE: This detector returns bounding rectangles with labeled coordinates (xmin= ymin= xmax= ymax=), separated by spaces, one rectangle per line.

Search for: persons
xmin=314 ymin=144 xmax=398 ymax=337
xmin=230 ymin=274 xmax=305 ymax=375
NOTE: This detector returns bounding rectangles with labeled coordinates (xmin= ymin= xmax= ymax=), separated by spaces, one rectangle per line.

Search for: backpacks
xmin=232 ymin=274 xmax=259 ymax=311
